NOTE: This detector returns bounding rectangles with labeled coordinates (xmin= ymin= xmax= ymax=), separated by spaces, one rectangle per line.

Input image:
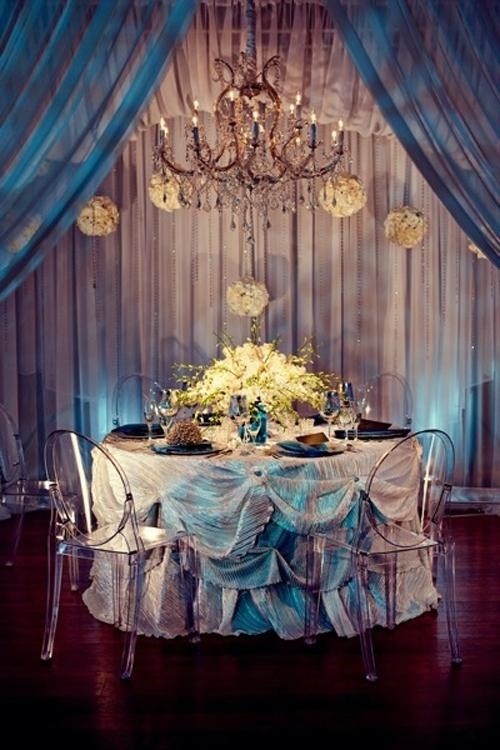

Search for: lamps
xmin=152 ymin=1 xmax=345 ymax=230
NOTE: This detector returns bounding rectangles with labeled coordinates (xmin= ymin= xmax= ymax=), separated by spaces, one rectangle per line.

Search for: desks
xmin=88 ymin=416 xmax=442 ymax=645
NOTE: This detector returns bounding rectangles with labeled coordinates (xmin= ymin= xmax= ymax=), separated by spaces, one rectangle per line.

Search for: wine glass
xmin=245 ymin=415 xmax=263 ymax=451
xmin=319 ymin=382 xmax=369 ymax=447
xmin=144 ymin=399 xmax=179 ymax=445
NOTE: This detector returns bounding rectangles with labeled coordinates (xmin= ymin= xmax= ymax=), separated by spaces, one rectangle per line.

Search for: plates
xmin=151 ymin=444 xmax=226 ymax=456
xmin=332 ymin=425 xmax=414 ymax=442
xmin=273 ymin=440 xmax=345 ymax=457
xmin=112 ymin=419 xmax=165 ymax=439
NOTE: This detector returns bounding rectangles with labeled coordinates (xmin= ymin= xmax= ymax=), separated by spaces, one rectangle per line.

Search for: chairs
xmin=362 ymin=373 xmax=416 ymax=430
xmin=110 ymin=374 xmax=166 ymax=428
xmin=36 ymin=428 xmax=205 ymax=679
xmin=302 ymin=428 xmax=462 ymax=678
xmin=0 ymin=406 xmax=84 ymax=595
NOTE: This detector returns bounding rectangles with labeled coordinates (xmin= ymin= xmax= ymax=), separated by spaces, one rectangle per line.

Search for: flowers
xmin=172 ymin=316 xmax=333 ymax=435
